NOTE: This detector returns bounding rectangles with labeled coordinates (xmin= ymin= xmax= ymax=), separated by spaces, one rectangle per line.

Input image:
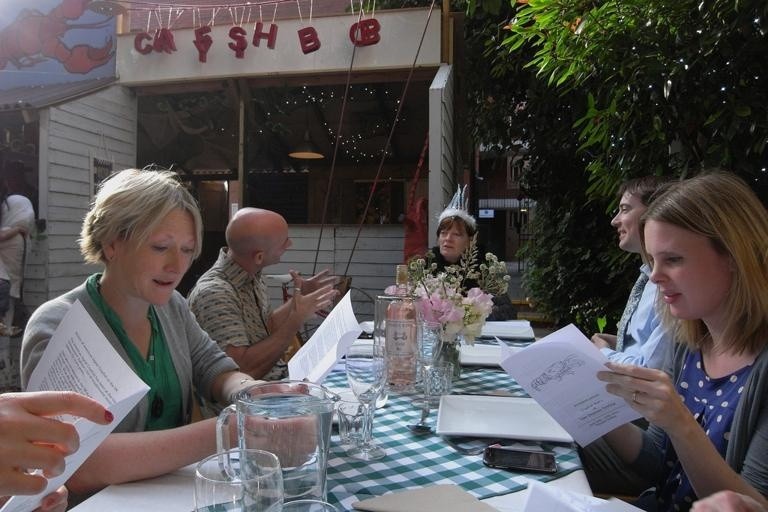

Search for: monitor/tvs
xmin=479 ymin=209 xmax=494 ymax=219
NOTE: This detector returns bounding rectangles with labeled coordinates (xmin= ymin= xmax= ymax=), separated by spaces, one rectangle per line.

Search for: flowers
xmin=385 ymin=231 xmax=511 ymax=346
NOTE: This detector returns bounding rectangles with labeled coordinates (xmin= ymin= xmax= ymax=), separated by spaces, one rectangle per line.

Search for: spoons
xmin=407 ymin=401 xmax=431 ymax=433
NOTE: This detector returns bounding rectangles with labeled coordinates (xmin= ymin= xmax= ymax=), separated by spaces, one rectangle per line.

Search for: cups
xmin=410 ymin=321 xmax=444 ymax=363
xmin=193 ymin=448 xmax=284 ymax=512
xmin=336 ymin=402 xmax=373 ymax=446
xmin=215 ymin=379 xmax=342 ymax=512
xmin=421 ymin=360 xmax=454 ymax=408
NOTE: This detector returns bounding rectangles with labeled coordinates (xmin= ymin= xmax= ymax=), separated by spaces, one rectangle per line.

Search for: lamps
xmin=288 ymin=104 xmax=324 ymax=159
xmin=520 ymin=198 xmax=528 ymax=212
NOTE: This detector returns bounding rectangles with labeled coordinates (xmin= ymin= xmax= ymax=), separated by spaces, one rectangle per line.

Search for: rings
xmin=294 ymin=287 xmax=301 ymax=291
xmin=630 ymin=391 xmax=640 ymax=401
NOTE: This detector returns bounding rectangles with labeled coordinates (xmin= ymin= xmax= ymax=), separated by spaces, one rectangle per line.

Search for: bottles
xmin=372 ymin=294 xmax=425 ymax=396
xmin=384 ymin=264 xmax=419 ymax=387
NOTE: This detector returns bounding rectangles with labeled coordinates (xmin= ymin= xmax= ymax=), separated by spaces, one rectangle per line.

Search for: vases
xmin=432 ymin=340 xmax=461 ymax=381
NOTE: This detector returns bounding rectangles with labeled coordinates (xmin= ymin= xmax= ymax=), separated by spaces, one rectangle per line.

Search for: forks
xmin=445 ymin=439 xmax=518 ymax=456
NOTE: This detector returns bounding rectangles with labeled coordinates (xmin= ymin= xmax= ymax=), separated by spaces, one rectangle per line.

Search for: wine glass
xmin=344 ymin=344 xmax=389 ymax=461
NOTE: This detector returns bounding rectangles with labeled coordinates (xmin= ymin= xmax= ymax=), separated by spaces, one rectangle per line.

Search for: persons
xmin=688 ymin=488 xmax=767 ymax=512
xmin=424 ymin=208 xmax=517 ymax=321
xmin=0 ymin=387 xmax=115 ymax=512
xmin=18 ymin=164 xmax=310 ymax=496
xmin=589 ymin=175 xmax=681 ymax=373
xmin=596 ymin=167 xmax=767 ymax=512
xmin=0 ymin=165 xmax=36 ymax=336
xmin=185 ymin=205 xmax=339 ymax=419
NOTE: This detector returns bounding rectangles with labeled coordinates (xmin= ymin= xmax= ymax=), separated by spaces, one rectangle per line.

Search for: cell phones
xmin=483 ymin=446 xmax=558 ymax=474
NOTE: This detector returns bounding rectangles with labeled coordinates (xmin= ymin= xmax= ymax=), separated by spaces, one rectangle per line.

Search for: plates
xmin=457 ymin=341 xmax=527 ymax=367
xmin=477 ymin=317 xmax=535 ymax=340
xmin=346 ymin=337 xmax=385 ymax=356
xmin=436 ymin=394 xmax=574 ymax=444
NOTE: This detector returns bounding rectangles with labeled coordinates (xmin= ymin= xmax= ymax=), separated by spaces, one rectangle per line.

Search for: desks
xmin=66 ymin=337 xmax=594 ymax=512
